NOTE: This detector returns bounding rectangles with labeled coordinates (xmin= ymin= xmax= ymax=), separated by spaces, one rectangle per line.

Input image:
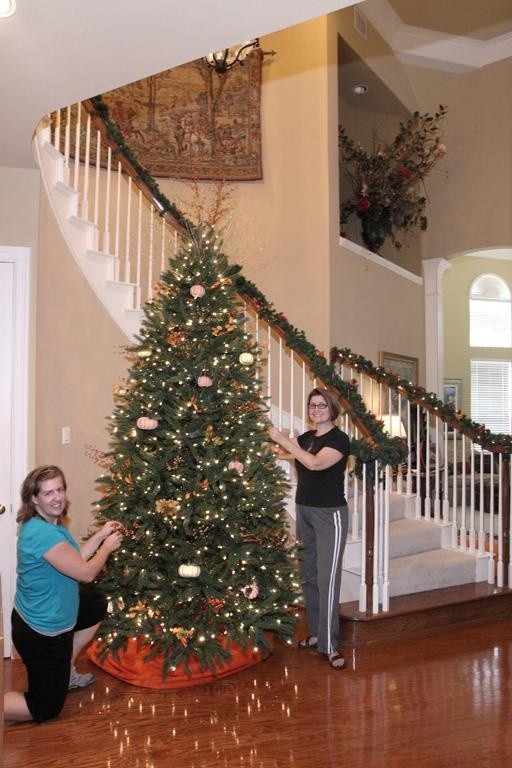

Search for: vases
xmin=362 ymin=222 xmax=387 ymax=256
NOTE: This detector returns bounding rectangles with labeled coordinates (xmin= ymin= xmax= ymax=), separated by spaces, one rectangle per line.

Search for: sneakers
xmin=69 ymin=667 xmax=96 ymax=690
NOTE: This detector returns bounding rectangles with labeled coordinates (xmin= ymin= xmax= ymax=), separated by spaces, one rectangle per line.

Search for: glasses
xmin=308 ymin=404 xmax=328 ymax=409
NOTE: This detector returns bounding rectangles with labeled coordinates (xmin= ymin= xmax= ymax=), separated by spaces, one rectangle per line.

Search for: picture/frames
xmin=443 ymin=378 xmax=466 ymax=440
xmin=378 ymin=349 xmax=419 ymax=416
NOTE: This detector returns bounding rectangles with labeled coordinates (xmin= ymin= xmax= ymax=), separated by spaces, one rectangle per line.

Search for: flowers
xmin=334 ymin=101 xmax=451 ymax=249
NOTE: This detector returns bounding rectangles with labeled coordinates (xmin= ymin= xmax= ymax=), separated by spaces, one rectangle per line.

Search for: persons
xmin=265 ymin=387 xmax=350 ymax=671
xmin=2 ymin=465 xmax=125 ymax=725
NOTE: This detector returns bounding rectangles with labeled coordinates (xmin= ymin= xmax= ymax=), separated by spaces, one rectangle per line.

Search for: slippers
xmin=325 ymin=651 xmax=345 ymax=670
xmin=298 ymin=635 xmax=317 ymax=649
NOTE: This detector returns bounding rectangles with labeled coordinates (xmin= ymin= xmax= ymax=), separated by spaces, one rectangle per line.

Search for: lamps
xmin=201 ymin=38 xmax=259 ymax=74
xmin=376 ymin=414 xmax=407 ymax=440
